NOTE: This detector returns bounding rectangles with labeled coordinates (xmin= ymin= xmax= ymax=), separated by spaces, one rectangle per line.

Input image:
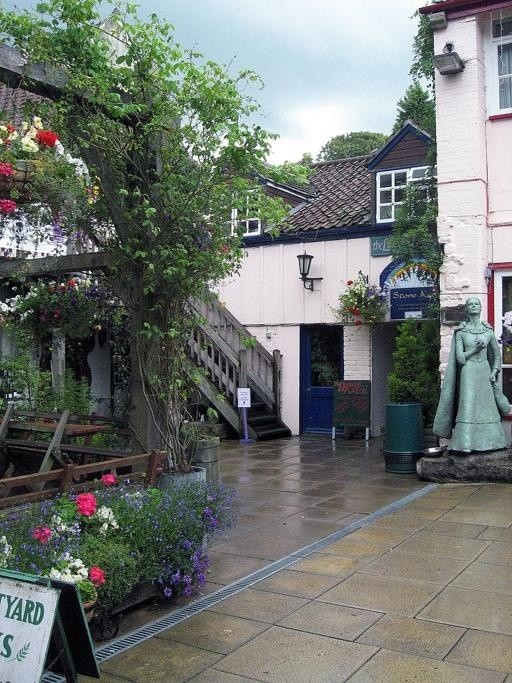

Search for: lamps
xmin=296 ymin=250 xmax=324 ymax=291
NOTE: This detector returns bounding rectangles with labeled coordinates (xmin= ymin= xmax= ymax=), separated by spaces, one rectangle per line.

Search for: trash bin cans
xmin=385 ymin=403 xmax=424 ymax=474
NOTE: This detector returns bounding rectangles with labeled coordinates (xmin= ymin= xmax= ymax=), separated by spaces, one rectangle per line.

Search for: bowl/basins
xmin=420 ymin=447 xmax=446 ymax=458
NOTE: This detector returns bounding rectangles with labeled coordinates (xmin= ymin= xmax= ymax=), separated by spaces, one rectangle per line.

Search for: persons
xmin=433 ymin=297 xmax=511 ymax=453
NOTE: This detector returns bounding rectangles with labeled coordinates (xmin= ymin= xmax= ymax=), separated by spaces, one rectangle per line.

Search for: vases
xmin=76 ymin=589 xmax=100 ymax=624
xmin=186 ymin=434 xmax=221 ymax=483
xmin=158 ymin=465 xmax=207 ymax=491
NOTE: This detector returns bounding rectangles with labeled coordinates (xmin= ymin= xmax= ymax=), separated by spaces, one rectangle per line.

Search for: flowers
xmin=325 ymin=274 xmax=393 ymax=334
xmin=0 ymin=471 xmax=243 ymax=606
xmin=0 ymin=98 xmax=244 ymax=348
